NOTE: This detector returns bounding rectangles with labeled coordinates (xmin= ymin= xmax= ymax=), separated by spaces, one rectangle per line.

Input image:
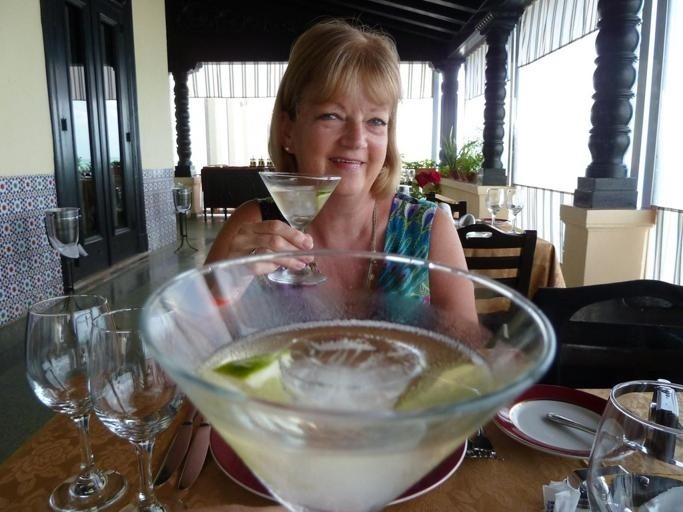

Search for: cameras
xmin=645 ymin=378 xmax=679 ymax=460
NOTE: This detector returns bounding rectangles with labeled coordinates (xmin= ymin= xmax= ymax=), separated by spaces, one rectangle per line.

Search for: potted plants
xmin=415 ymin=159 xmax=436 ymax=174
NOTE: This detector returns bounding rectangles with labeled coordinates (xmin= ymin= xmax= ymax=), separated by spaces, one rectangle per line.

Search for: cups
xmin=586 ymin=379 xmax=682 ymax=511
xmin=138 ymin=248 xmax=559 ymax=510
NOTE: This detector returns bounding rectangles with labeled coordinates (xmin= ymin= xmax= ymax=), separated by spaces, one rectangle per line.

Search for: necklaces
xmin=365 ymin=204 xmax=379 ymax=291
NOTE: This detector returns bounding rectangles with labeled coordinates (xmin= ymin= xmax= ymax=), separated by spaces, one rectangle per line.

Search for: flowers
xmin=415 ymin=171 xmax=442 ymax=194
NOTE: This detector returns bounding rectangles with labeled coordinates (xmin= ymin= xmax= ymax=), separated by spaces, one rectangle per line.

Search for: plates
xmin=209 ymin=426 xmax=468 ymax=507
xmin=491 ymin=383 xmax=646 ymax=461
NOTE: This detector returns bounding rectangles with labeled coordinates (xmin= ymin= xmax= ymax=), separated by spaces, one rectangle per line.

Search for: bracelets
xmin=206 ymin=293 xmax=234 ymax=308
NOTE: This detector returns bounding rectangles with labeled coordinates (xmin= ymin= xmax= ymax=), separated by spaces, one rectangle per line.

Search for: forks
xmin=467 ymin=424 xmax=504 ymax=464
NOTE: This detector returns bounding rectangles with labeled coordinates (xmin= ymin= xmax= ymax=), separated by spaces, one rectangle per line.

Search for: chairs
xmin=427 ymin=196 xmax=466 ymax=221
xmin=454 ymin=223 xmax=683 ymax=383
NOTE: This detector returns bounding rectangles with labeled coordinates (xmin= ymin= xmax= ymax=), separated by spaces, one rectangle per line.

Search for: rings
xmin=248 ymin=246 xmax=256 ymax=258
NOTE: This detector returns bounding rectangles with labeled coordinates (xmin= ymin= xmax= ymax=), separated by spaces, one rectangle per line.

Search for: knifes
xmin=152 ymin=401 xmax=212 ymax=491
xmin=547 ymin=411 xmax=682 ymax=468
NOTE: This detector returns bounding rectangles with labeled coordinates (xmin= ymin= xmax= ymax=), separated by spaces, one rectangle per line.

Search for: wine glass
xmin=486 ymin=187 xmax=525 ymax=235
xmin=24 ymin=293 xmax=129 ymax=511
xmin=88 ymin=306 xmax=192 ymax=512
xmin=258 ymin=169 xmax=343 ymax=287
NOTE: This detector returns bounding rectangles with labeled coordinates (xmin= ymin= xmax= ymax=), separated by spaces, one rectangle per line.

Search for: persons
xmin=201 ymin=20 xmax=482 ymax=352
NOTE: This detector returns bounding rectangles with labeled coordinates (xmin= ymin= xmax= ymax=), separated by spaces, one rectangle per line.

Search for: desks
xmin=0 ymin=350 xmax=682 ymax=512
xmin=200 ymin=165 xmax=277 ymax=225
xmin=452 ymin=218 xmax=556 ymax=316
